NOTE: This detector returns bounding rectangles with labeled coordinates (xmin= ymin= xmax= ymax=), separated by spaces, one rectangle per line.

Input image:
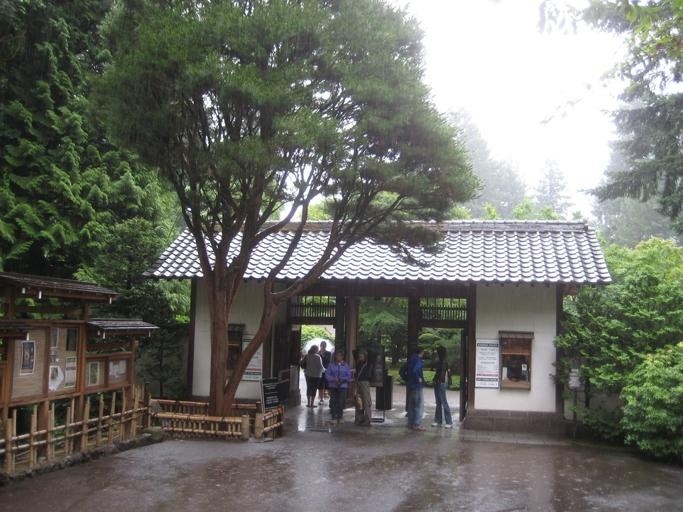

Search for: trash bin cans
xmin=375 ymin=376 xmax=392 ymax=410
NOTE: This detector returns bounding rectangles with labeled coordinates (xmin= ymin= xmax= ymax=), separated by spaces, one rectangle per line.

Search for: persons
xmin=431 ymin=345 xmax=454 ymax=428
xmin=325 ymin=349 xmax=351 ymax=424
xmin=353 ymin=348 xmax=373 ymax=426
xmin=399 ymin=346 xmax=426 ymax=431
xmin=302 ymin=345 xmax=327 ymax=408
xmin=317 ymin=341 xmax=331 ymax=405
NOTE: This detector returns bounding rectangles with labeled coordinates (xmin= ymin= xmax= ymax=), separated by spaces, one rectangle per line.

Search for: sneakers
xmin=411 ymin=422 xmax=451 ymax=431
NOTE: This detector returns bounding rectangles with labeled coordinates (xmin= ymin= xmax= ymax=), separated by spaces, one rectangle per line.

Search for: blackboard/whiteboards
xmin=262 ymin=379 xmax=279 ymax=407
